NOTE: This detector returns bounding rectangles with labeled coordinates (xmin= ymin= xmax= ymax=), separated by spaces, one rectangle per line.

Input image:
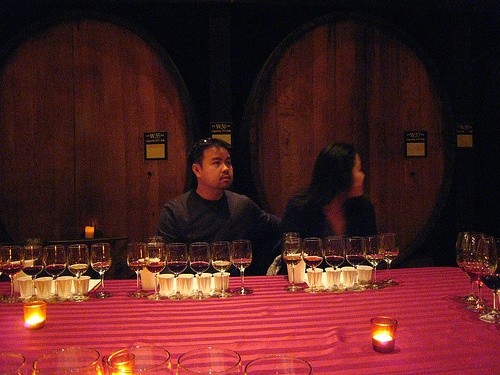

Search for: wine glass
xmin=0 ymin=243 xmax=112 ymax=303
xmin=456 ymin=231 xmax=500 ymax=323
xmin=126 ymin=234 xmax=252 ymax=301
xmin=283 ymin=232 xmax=399 ymax=294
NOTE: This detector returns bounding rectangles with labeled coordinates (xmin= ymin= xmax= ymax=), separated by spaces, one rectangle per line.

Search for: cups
xmin=0 ymin=344 xmax=313 ymax=375
xmin=23 ymin=300 xmax=46 ymax=330
xmin=370 ymin=316 xmax=398 ymax=353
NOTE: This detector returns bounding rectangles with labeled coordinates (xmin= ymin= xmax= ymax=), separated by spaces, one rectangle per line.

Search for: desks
xmin=0 ymin=267 xmax=500 ymax=375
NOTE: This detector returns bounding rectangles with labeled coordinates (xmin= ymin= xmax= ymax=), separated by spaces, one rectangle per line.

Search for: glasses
xmin=195 ymin=138 xmax=213 ymax=149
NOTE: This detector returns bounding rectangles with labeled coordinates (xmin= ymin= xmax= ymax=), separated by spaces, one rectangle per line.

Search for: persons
xmin=156 ymin=138 xmax=282 ymax=277
xmin=281 ymin=142 xmax=377 ymax=268
